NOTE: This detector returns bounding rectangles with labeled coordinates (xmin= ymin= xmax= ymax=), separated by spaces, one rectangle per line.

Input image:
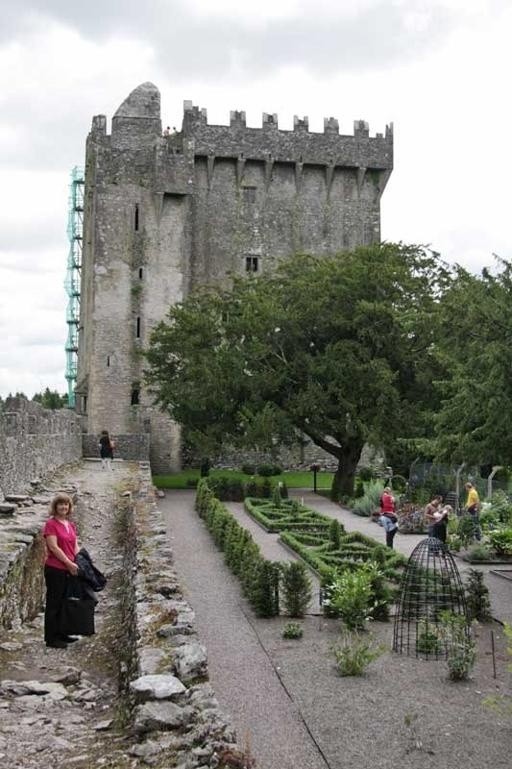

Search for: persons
xmin=425 ymin=494 xmax=442 ymax=535
xmin=98 ymin=429 xmax=114 ymax=469
xmin=462 ymin=482 xmax=480 ymax=532
xmin=42 ymin=491 xmax=80 ymax=648
xmin=432 ymin=504 xmax=453 ymax=544
xmin=379 ymin=486 xmax=394 ymax=519
xmin=372 ymin=510 xmax=399 ymax=550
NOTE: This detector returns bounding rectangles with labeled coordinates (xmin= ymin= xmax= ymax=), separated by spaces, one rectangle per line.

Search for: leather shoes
xmin=46 ymin=641 xmax=67 ymax=648
xmin=61 ymin=636 xmax=79 ymax=642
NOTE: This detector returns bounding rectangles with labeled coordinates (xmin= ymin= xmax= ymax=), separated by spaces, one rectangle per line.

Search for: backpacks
xmin=382 ymin=511 xmax=398 ymax=523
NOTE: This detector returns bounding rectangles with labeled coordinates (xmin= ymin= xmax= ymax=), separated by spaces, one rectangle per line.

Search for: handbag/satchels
xmin=58 ymin=575 xmax=96 ymax=635
xmin=380 ymin=496 xmax=383 ymax=508
